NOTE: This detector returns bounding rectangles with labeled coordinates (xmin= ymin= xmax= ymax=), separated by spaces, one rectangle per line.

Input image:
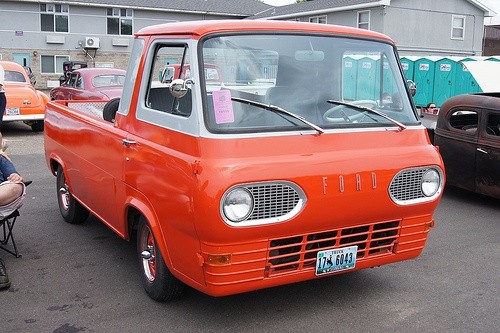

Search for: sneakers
xmin=0 ymin=257 xmax=11 ymax=288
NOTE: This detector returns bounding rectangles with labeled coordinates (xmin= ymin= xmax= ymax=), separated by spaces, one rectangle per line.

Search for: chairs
xmin=150 ymin=87 xmax=192 ymax=114
xmin=265 ymin=85 xmax=319 ymax=126
xmin=0 ymin=180 xmax=35 ymax=258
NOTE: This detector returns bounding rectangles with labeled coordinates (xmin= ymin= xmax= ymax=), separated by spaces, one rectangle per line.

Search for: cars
xmin=0 ymin=60 xmax=51 ymax=132
xmin=50 ymin=66 xmax=128 ymax=101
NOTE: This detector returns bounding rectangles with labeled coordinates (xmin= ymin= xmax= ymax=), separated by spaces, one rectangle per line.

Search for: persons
xmin=0 ymin=65 xmax=26 ymax=292
xmin=390 ymin=94 xmax=403 ymax=108
xmin=425 ymin=103 xmax=436 ymax=114
xmin=384 ymin=93 xmax=393 ymax=101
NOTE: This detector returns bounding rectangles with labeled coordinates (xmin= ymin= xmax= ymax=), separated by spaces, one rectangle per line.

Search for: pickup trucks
xmin=421 ymin=90 xmax=500 ymax=201
xmin=158 ymin=61 xmax=275 ymax=95
xmin=42 ymin=16 xmax=449 ymax=307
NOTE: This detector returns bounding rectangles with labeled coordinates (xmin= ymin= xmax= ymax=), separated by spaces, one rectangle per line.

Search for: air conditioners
xmin=86 ymin=37 xmax=100 ymax=47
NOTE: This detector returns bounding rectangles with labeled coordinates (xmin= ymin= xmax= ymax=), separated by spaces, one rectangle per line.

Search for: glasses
xmin=429 ymin=107 xmax=436 ymax=109
